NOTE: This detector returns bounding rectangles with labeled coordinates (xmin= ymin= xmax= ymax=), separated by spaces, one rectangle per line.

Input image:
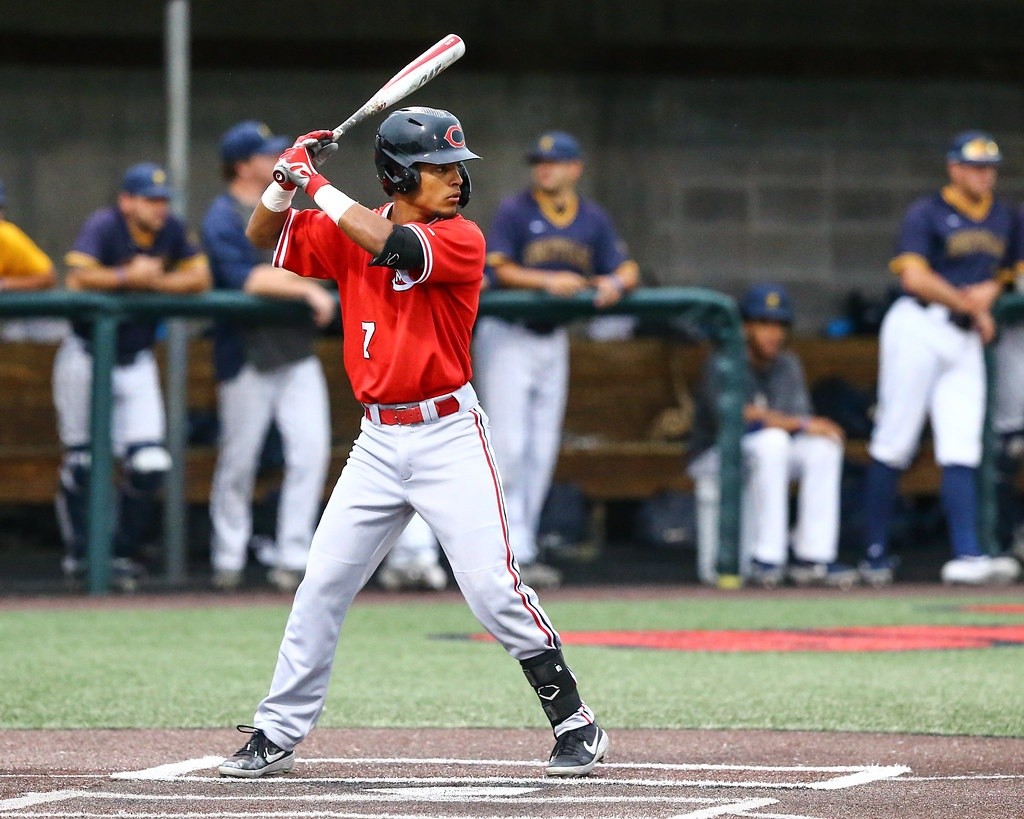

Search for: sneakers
xmin=862 ymin=555 xmax=893 ymax=587
xmin=546 ymin=721 xmax=609 ymax=777
xmin=268 ymin=567 xmax=301 ymax=597
xmin=212 ymin=568 xmax=245 ymax=591
xmin=751 ymin=563 xmax=787 ymax=587
xmin=515 ymin=561 xmax=562 ymax=595
xmin=218 ymin=725 xmax=296 ymax=778
xmin=939 ymin=553 xmax=1018 ymax=588
xmin=65 ymin=557 xmax=152 ymax=593
xmin=789 ymin=561 xmax=858 ymax=587
xmin=380 ymin=562 xmax=444 ymax=593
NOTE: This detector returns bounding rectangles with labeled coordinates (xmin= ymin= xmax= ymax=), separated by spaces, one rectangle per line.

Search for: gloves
xmin=272 ymin=130 xmax=338 ymax=201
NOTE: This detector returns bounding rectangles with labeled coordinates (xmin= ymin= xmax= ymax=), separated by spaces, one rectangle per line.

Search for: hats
xmin=524 ymin=132 xmax=587 ymax=164
xmin=739 ymin=283 xmax=796 ymax=325
xmin=223 ymin=120 xmax=294 ymax=159
xmin=120 ymin=162 xmax=175 ymax=200
xmin=947 ymin=129 xmax=1004 ymax=164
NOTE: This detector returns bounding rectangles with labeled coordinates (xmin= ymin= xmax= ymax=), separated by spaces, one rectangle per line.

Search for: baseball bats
xmin=272 ymin=31 xmax=467 ymax=184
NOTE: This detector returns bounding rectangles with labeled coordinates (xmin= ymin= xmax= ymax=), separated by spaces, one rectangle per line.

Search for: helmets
xmin=375 ymin=107 xmax=481 ymax=208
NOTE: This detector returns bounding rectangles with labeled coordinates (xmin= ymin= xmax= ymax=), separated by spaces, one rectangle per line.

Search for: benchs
xmin=0 ymin=311 xmax=941 ymax=498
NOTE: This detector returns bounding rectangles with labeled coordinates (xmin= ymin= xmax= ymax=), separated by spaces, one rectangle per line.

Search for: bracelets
xmin=261 ymin=179 xmax=298 ymax=212
xmin=314 ymin=183 xmax=359 ymax=227
xmin=605 ymin=272 xmax=627 ymax=293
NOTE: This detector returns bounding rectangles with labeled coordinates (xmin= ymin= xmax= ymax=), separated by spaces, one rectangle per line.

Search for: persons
xmin=0 ymin=215 xmax=60 ymax=293
xmin=50 ymin=161 xmax=211 ymax=593
xmin=254 ymin=265 xmax=694 ymax=591
xmin=468 ymin=128 xmax=641 ymax=590
xmin=685 ymin=282 xmax=860 ymax=589
xmin=198 ymin=119 xmax=337 ymax=596
xmin=218 ymin=105 xmax=612 ymax=781
xmin=853 ymin=127 xmax=1024 ymax=590
